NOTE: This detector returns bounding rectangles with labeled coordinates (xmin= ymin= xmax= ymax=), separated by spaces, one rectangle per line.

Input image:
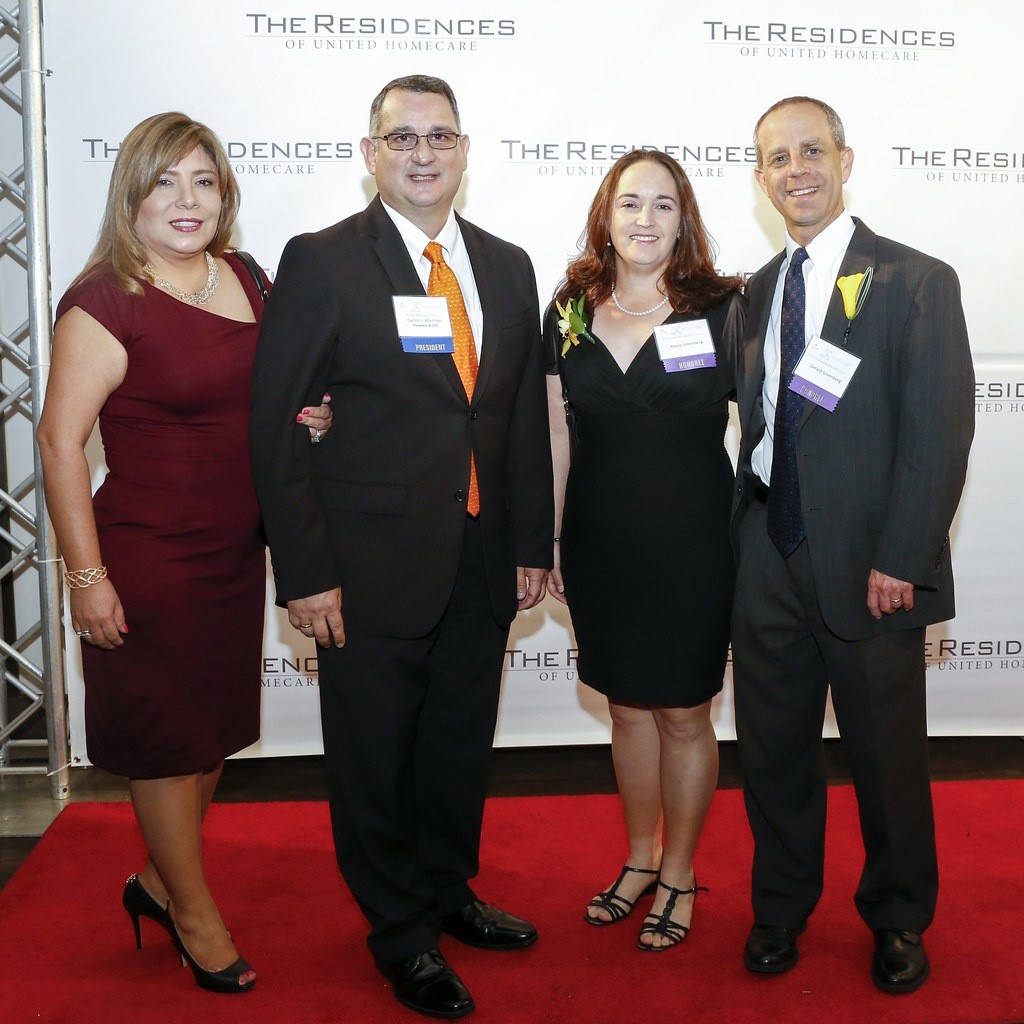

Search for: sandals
xmin=634 ymin=872 xmax=709 ymax=951
xmin=582 ymin=867 xmax=663 ymax=926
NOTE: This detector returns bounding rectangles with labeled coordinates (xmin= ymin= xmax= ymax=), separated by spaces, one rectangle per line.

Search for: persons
xmin=251 ymin=73 xmax=557 ymax=1019
xmin=698 ymin=96 xmax=975 ymax=995
xmin=36 ymin=112 xmax=333 ymax=993
xmin=544 ymin=152 xmax=752 ymax=952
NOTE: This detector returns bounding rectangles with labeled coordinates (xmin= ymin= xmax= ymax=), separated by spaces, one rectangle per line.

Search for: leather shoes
xmin=871 ymin=931 xmax=930 ymax=995
xmin=376 ymin=944 xmax=475 ymax=1021
xmin=743 ymin=915 xmax=808 ymax=976
xmin=439 ymin=880 xmax=539 ymax=952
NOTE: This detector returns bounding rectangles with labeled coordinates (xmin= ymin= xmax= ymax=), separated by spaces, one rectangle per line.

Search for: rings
xmin=314 ymin=429 xmax=322 ymax=443
xmin=82 ymin=630 xmax=90 ymax=636
xmin=77 ymin=632 xmax=82 ymax=637
xmin=300 ymin=623 xmax=312 ymax=628
xmin=892 ymin=597 xmax=901 ymax=603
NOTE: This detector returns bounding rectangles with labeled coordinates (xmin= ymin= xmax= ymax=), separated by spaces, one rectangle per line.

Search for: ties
xmin=765 ymin=248 xmax=810 ymax=561
xmin=421 ymin=242 xmax=481 ymax=518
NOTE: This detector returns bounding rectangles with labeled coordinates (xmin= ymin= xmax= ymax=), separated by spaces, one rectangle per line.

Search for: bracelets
xmin=64 ymin=565 xmax=107 ymax=589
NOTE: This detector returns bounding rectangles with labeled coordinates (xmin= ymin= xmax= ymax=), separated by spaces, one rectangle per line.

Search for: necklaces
xmin=610 ymin=280 xmax=669 ymax=317
xmin=137 ymin=252 xmax=219 ymax=305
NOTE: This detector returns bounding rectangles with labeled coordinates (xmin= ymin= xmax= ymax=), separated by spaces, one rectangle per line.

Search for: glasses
xmin=372 ymin=131 xmax=460 ymax=151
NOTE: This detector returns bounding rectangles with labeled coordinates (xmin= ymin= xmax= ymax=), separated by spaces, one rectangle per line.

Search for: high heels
xmin=122 ymin=873 xmax=169 ymax=951
xmin=161 ymin=899 xmax=255 ymax=994
